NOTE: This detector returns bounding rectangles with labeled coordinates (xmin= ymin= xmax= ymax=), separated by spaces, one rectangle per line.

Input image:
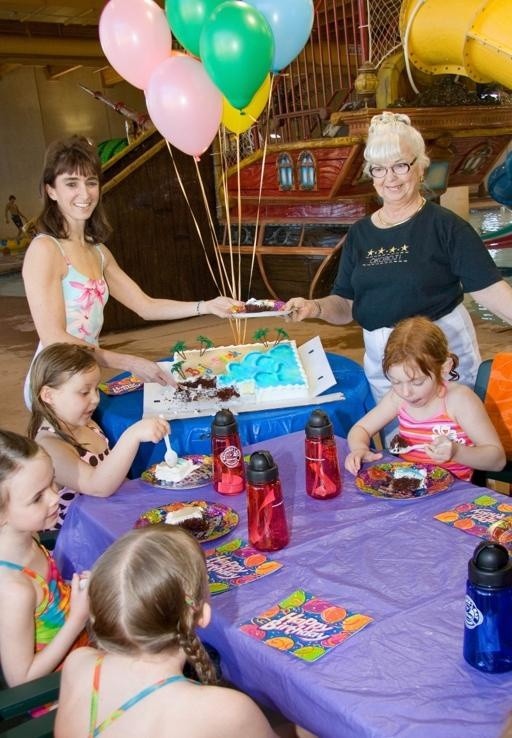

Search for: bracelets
xmin=196 ymin=300 xmax=204 ymax=316
xmin=312 ymin=297 xmax=322 ymax=319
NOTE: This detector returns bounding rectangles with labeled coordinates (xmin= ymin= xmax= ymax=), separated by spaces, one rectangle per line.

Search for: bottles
xmin=459 ymin=538 xmax=512 ymax=675
xmin=243 ymin=449 xmax=290 ymax=553
xmin=299 ymin=408 xmax=344 ymax=500
xmin=207 ymin=407 xmax=246 ymax=497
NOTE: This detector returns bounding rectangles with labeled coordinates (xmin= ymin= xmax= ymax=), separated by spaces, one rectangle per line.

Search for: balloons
xmin=97 ymin=0 xmax=315 ymax=163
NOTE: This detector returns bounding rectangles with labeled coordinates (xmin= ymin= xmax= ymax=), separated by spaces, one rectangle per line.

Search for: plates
xmin=140 ymin=452 xmax=215 ymax=491
xmin=133 ymin=499 xmax=239 ymax=543
xmin=227 ymin=299 xmax=294 ymax=319
xmin=354 ymin=463 xmax=455 ymax=503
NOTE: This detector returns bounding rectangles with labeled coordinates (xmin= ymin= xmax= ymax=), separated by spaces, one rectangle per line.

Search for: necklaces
xmin=377 ymin=197 xmax=426 ymax=226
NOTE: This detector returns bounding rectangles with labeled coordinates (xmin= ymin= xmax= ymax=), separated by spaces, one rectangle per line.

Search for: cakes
xmin=166 ymin=506 xmax=207 ymax=534
xmin=390 ymin=462 xmax=427 ymax=492
xmin=154 ymin=457 xmax=198 ymax=482
xmin=231 ymin=297 xmax=286 ymax=313
xmin=389 ymin=433 xmax=406 ymax=449
xmin=170 ymin=328 xmax=309 ymax=402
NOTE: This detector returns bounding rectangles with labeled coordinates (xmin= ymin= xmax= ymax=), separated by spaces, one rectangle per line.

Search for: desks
xmin=69 ymin=420 xmax=511 ymax=738
xmin=90 ymin=341 xmax=377 ymax=478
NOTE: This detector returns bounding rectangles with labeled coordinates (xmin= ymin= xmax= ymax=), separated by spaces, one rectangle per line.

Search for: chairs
xmin=0 ymin=699 xmax=73 ymax=736
xmin=3 ymin=665 xmax=67 ymax=718
xmin=471 ymin=354 xmax=511 ymax=497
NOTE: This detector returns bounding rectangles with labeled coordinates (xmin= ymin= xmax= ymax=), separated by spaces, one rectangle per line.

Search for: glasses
xmin=369 ymin=156 xmax=418 ymax=178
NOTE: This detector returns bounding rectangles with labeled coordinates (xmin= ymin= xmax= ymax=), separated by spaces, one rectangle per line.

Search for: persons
xmin=23 ymin=340 xmax=173 ymax=533
xmin=51 ymin=522 xmax=318 ymax=738
xmin=1 ymin=426 xmax=94 ymax=722
xmin=276 ymin=108 xmax=512 ymax=412
xmin=21 ymin=132 xmax=244 ymax=417
xmin=4 ymin=193 xmax=29 ymax=245
xmin=340 ymin=313 xmax=507 ymax=475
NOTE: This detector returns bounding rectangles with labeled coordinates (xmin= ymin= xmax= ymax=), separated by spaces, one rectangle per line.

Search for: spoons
xmin=156 ymin=414 xmax=178 ymax=467
xmin=387 ymin=443 xmax=427 ymax=456
